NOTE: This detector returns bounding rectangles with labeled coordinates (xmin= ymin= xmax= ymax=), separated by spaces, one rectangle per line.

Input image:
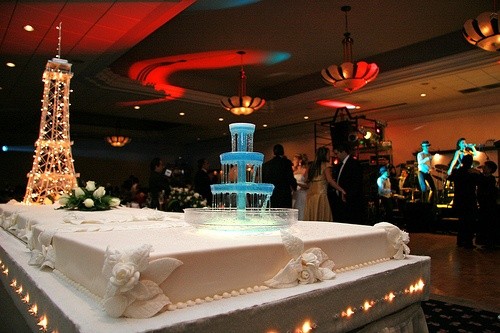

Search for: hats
xmin=379 ymin=166 xmax=389 ymax=173
xmin=420 ymin=140 xmax=431 ymax=147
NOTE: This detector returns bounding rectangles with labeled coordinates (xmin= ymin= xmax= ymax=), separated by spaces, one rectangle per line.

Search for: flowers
xmin=48 ymin=180 xmax=122 ymax=212
xmin=373 ymin=221 xmax=411 ymax=260
xmin=261 ymin=228 xmax=337 ymax=288
xmin=0 ymin=210 xmax=57 ymax=271
xmin=102 ymin=241 xmax=185 ymax=320
xmin=164 ymin=186 xmax=211 ymax=212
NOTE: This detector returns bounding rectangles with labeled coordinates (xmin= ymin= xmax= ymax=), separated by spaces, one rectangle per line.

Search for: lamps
xmin=462 ymin=0 xmax=500 ymax=52
xmin=103 ymin=115 xmax=132 ymax=148
xmin=219 ymin=51 xmax=266 ymax=116
xmin=320 ymin=6 xmax=379 ymax=93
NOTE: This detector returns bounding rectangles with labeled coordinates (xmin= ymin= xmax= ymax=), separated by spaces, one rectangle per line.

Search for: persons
xmin=99 ymin=138 xmax=500 ymax=250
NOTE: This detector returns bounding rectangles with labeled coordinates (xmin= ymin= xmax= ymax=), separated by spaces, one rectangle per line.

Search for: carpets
xmin=420 ymin=293 xmax=500 ymax=333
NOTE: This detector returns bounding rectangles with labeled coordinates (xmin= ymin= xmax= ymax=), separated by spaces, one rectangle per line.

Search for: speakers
xmin=331 ymin=121 xmax=357 ymax=151
